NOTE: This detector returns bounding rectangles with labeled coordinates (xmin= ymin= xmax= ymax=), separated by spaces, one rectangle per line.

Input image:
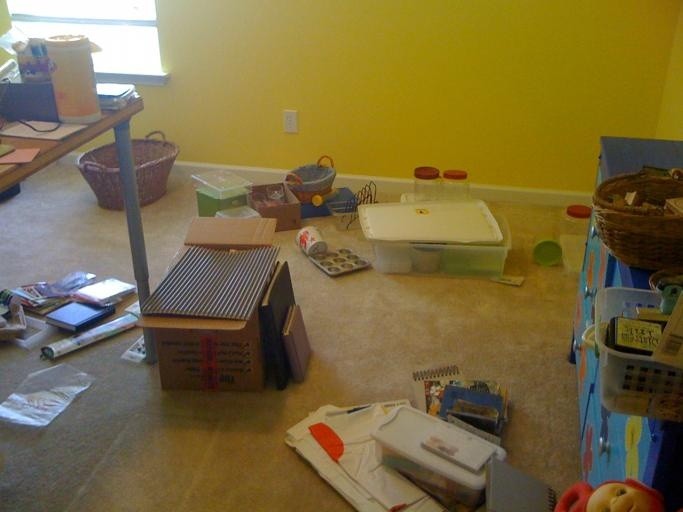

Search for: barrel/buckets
xmin=43 ymin=35 xmax=102 ymax=124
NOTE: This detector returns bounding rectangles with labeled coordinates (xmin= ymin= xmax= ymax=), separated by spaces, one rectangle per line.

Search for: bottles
xmin=441 ymin=170 xmax=470 ymax=203
xmin=410 ymin=166 xmax=442 ymax=204
xmin=560 ymin=203 xmax=592 ymax=274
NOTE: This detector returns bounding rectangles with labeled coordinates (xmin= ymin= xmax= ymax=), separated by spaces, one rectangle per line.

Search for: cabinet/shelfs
xmin=568 ymin=135 xmax=682 ymax=511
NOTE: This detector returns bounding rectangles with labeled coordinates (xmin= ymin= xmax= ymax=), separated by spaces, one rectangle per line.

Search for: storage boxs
xmin=134 ymin=215 xmax=278 ymax=394
xmin=366 ymin=403 xmax=508 ymax=512
xmin=243 ymin=181 xmax=302 ymax=231
xmin=356 ymin=198 xmax=513 ymax=279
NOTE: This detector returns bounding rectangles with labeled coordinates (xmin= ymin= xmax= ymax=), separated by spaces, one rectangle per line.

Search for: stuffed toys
xmin=554 ymin=477 xmax=664 ymax=511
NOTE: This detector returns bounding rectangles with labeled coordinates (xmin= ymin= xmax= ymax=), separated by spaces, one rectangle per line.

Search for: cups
xmin=266 ymin=184 xmax=286 ymax=203
xmin=294 ymin=224 xmax=328 ymax=256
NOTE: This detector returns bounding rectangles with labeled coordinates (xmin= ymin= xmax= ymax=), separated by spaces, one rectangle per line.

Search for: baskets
xmin=285 ymin=155 xmax=336 ymax=203
xmin=594 ymin=286 xmax=683 ymax=424
xmin=77 ymin=131 xmax=180 ymax=209
xmin=593 ymin=169 xmax=682 ymax=272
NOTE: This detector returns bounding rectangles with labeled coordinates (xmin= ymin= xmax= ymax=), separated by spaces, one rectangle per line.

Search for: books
xmin=281 ymin=302 xmax=313 ymax=383
xmin=258 ymin=260 xmax=296 ymax=391
xmin=45 ymin=302 xmax=115 ymax=333
xmin=483 ymin=457 xmax=556 ymax=512
